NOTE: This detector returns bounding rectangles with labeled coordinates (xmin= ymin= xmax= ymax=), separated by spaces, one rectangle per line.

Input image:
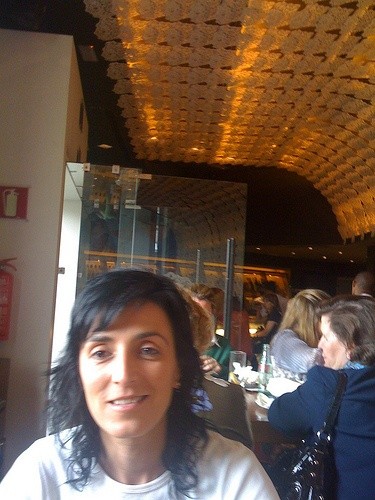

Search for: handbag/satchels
xmin=267 ymin=373 xmax=347 ymax=500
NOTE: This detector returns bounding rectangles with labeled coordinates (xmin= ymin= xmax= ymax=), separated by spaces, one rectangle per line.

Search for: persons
xmin=251 ymin=295 xmax=282 ymax=342
xmin=269 ymin=288 xmax=334 ymax=381
xmin=219 ymin=295 xmax=258 ymax=372
xmin=181 ymin=282 xmax=231 ymax=382
xmin=351 ymin=272 xmax=375 ymax=297
xmin=267 ymin=293 xmax=375 ymax=499
xmin=1 ymin=269 xmax=280 ymax=499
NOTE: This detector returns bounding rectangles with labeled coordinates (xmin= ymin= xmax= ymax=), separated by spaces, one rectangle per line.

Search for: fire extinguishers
xmin=2 ymin=187 xmax=19 ymax=218
xmin=0 ymin=257 xmax=18 ymax=342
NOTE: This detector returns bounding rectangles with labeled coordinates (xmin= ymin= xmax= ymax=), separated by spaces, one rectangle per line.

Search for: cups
xmin=228 ymin=350 xmax=246 ymax=385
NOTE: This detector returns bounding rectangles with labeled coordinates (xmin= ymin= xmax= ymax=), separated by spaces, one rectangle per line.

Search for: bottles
xmin=258 ymin=343 xmax=273 ymax=396
